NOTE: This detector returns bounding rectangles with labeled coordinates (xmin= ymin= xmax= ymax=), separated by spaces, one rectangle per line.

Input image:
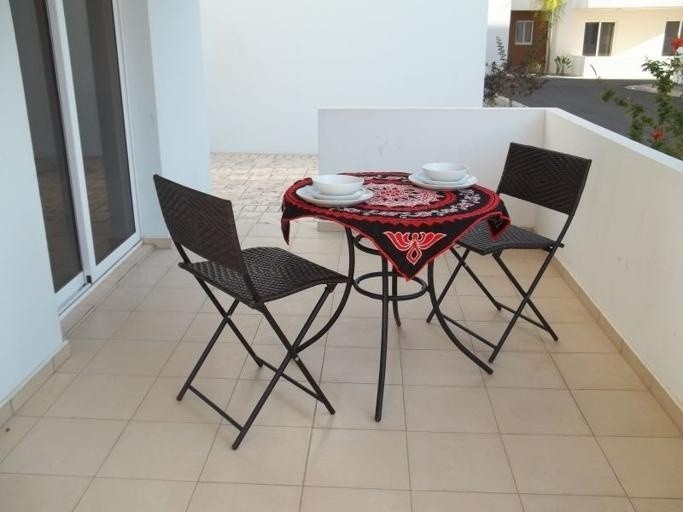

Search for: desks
xmin=280 ymin=171 xmax=511 ymax=422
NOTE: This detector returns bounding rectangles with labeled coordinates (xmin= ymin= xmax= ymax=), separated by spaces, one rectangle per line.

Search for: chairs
xmin=153 ymin=174 xmax=355 ymax=449
xmin=426 ymin=142 xmax=592 ymax=363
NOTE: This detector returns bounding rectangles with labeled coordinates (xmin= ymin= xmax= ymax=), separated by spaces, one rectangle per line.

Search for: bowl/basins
xmin=316 ymin=173 xmax=364 ymax=195
xmin=422 ymin=162 xmax=469 ymax=182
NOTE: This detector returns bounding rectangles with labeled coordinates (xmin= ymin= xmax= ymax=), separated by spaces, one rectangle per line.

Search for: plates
xmin=409 ymin=174 xmax=478 ymax=190
xmin=306 ymin=185 xmax=372 ymax=198
xmin=415 ymin=173 xmax=478 ymax=185
xmin=296 ymin=186 xmax=373 ymax=208
